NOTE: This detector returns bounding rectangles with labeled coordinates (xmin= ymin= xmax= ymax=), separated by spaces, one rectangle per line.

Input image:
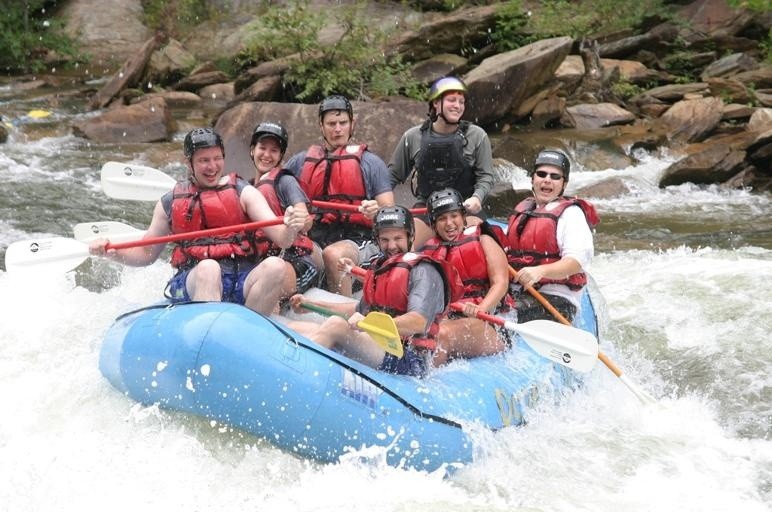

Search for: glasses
xmin=534 ymin=170 xmax=565 ymax=180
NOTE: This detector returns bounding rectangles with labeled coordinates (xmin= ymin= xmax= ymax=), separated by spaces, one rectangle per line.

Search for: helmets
xmin=426 ymin=186 xmax=466 ymax=225
xmin=319 ymin=95 xmax=353 ymax=120
xmin=250 ymin=121 xmax=289 ymax=153
xmin=530 ymin=148 xmax=570 ymax=182
xmin=428 ymin=76 xmax=468 ymax=105
xmin=372 ymin=204 xmax=415 ymax=244
xmin=182 ymin=127 xmax=226 ymax=161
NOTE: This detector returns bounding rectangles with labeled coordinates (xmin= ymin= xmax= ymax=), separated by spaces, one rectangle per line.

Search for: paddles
xmin=4 ymin=216 xmax=287 ymax=273
xmin=74 ymin=221 xmax=177 ymax=251
xmin=300 ymin=301 xmax=404 ymax=358
xmin=101 ymin=162 xmax=363 ymax=214
xmin=450 ymin=300 xmax=598 ymax=371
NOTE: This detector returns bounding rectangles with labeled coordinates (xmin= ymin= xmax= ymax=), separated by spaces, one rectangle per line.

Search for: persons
xmin=283 ymin=94 xmax=396 ymax=298
xmin=89 ymin=128 xmax=308 ymax=316
xmin=288 ymin=205 xmax=465 ymax=380
xmin=337 ymin=186 xmax=513 ymax=368
xmin=504 ymin=150 xmax=600 ymax=324
xmin=246 ymin=119 xmax=319 ymax=316
xmin=388 ymin=77 xmax=497 ymax=253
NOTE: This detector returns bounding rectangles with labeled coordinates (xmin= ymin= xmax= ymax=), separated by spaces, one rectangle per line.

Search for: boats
xmin=100 ymin=271 xmax=605 ymax=478
